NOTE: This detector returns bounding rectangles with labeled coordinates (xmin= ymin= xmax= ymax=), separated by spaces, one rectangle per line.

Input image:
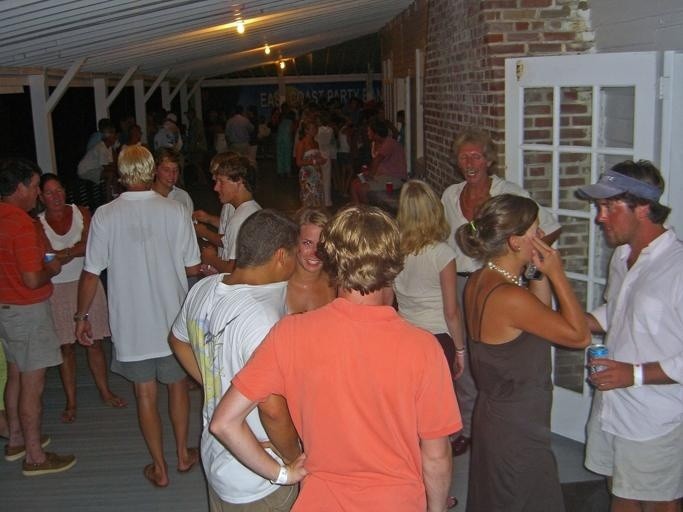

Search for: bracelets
xmin=630 ymin=362 xmax=645 ymax=388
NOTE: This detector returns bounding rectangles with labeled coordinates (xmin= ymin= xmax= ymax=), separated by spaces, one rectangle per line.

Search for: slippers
xmin=62 ymin=409 xmax=75 ymax=423
xmin=178 ymin=448 xmax=201 ymax=473
xmin=144 ymin=464 xmax=169 ymax=487
xmin=103 ymin=396 xmax=128 ymax=408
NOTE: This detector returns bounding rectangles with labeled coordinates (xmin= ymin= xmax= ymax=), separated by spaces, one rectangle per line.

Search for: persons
xmin=168 ymin=209 xmax=304 ymax=512
xmin=72 ymin=145 xmax=202 ymax=490
xmin=193 ymin=151 xmax=263 ymax=271
xmin=574 ymin=159 xmax=683 ymax=512
xmin=75 ymin=108 xmax=229 ymax=198
xmin=209 ymin=200 xmax=464 ymax=512
xmin=454 ymin=193 xmax=591 ymax=511
xmin=383 ymin=178 xmax=469 ymax=511
xmin=230 ymin=96 xmax=406 ymax=206
xmin=0 ymin=157 xmax=126 ymax=471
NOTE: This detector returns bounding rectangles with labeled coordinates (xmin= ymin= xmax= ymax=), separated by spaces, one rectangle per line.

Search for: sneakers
xmin=23 ymin=452 xmax=77 ymax=477
xmin=4 ymin=434 xmax=52 ymax=461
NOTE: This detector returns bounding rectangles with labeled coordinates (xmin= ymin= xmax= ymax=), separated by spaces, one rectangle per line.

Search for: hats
xmin=577 ymin=169 xmax=662 ymax=203
